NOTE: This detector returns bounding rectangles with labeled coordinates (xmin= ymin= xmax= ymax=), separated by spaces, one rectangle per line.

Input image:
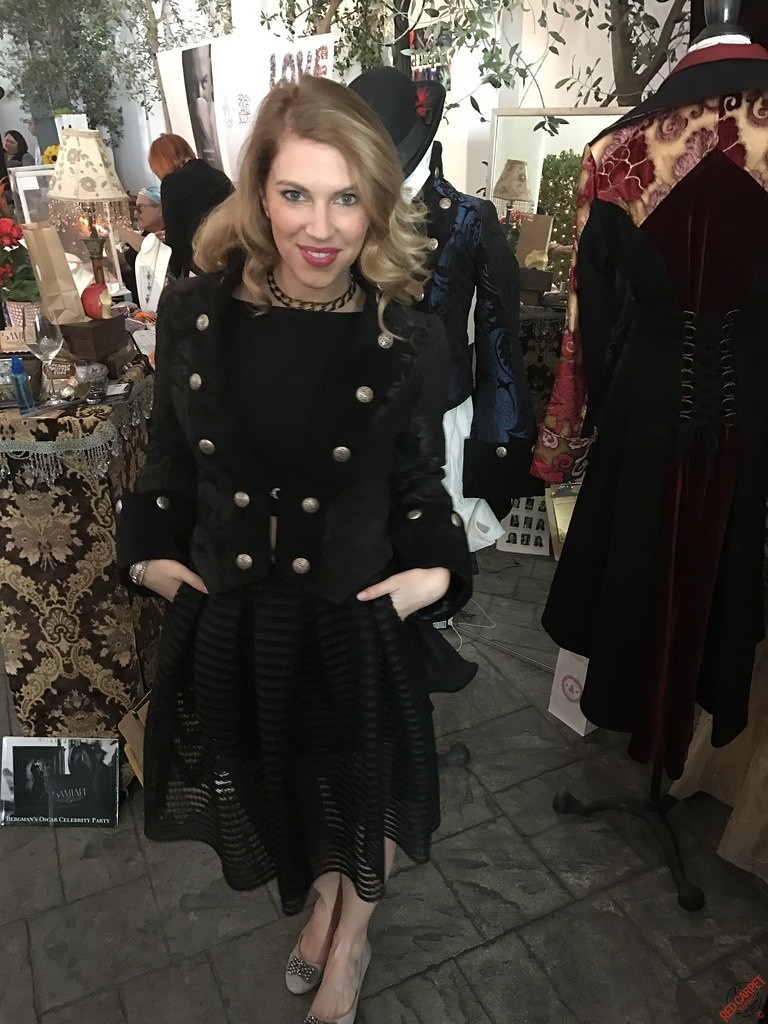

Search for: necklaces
xmin=140 ymin=240 xmax=160 ymax=303
xmin=267 ymin=265 xmax=357 ymax=312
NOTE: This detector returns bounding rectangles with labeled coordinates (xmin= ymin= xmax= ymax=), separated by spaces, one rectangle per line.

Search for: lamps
xmin=46 ymin=129 xmax=132 ymax=283
xmin=493 ymin=158 xmax=535 ymax=229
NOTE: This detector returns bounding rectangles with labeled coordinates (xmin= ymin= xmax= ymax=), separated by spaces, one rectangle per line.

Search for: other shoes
xmin=303 ymin=937 xmax=372 ymax=1024
xmin=285 ymin=913 xmax=327 ymax=994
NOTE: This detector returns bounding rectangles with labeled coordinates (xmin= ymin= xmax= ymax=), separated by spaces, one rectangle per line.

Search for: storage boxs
xmin=59 ymin=311 xmax=128 ymax=361
xmin=547 ymin=647 xmax=599 ymax=736
xmin=98 ymin=335 xmax=139 ymax=379
xmin=518 ymin=265 xmax=553 ymax=306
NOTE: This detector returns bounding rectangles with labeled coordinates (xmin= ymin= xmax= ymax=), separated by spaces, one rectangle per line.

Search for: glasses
xmin=132 ymin=203 xmax=158 ymax=214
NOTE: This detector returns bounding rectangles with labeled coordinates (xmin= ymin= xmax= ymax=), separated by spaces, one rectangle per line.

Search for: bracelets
xmin=129 ymin=559 xmax=148 ymax=587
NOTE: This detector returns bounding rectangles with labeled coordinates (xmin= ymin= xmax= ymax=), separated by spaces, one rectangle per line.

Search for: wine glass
xmin=22 ymin=305 xmax=64 ymax=410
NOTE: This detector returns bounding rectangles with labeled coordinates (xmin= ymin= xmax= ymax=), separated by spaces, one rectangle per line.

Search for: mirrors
xmin=486 ymin=106 xmax=637 ymax=306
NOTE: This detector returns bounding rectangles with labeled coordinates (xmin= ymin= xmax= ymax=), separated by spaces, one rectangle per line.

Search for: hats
xmin=346 ymin=66 xmax=446 ymax=180
xmin=139 ymin=186 xmax=162 ymax=207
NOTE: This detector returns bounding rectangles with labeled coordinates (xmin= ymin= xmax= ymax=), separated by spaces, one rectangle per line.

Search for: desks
xmin=1 ymin=354 xmax=166 ymax=808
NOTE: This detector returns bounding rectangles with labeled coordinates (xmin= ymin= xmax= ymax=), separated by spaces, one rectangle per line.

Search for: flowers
xmin=0 ymin=217 xmax=42 ymax=303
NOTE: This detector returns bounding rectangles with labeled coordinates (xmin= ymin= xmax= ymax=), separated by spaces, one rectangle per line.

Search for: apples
xmin=525 ymin=248 xmax=548 ymax=271
xmin=81 ymin=282 xmax=113 ymax=319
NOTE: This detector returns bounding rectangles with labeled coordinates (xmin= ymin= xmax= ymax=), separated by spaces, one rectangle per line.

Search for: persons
xmin=111 ymin=75 xmax=478 ymax=1024
xmin=118 ymin=186 xmax=183 ymax=309
xmin=347 ymin=66 xmax=536 ymax=558
xmin=147 ymin=133 xmax=240 ymax=279
xmin=526 ymin=19 xmax=767 ymax=779
xmin=3 ymin=130 xmax=36 ymax=168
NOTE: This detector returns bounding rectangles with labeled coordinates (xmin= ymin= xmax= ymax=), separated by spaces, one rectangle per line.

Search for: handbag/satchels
xmin=21 ymin=221 xmax=94 ymax=325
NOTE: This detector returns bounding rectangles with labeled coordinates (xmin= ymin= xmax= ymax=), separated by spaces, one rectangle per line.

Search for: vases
xmin=5 ymin=300 xmax=39 ymax=326
